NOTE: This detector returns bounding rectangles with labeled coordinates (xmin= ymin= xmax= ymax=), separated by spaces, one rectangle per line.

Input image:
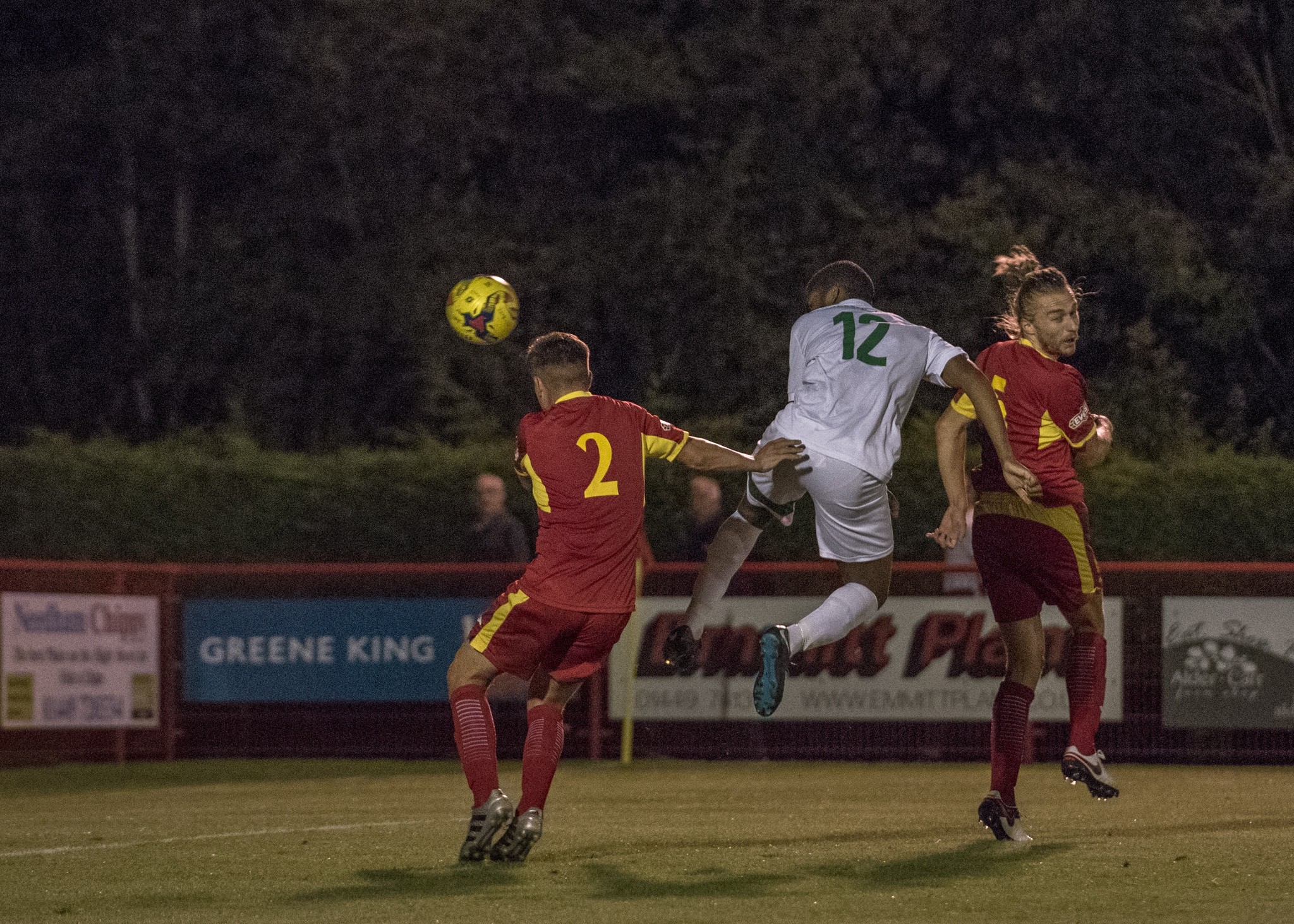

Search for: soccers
xmin=446 ymin=274 xmax=520 ymax=347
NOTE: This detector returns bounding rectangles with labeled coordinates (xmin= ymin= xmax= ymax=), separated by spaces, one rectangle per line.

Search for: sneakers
xmin=1063 ymin=748 xmax=1119 ymax=801
xmin=977 ymin=791 xmax=1034 ymax=841
xmin=753 ymin=625 xmax=792 ymax=717
xmin=491 ymin=806 xmax=544 ymax=863
xmin=661 ymin=625 xmax=704 ymax=676
xmin=458 ymin=786 xmax=515 ymax=860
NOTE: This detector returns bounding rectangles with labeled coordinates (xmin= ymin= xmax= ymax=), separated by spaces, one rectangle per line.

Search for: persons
xmin=924 ymin=244 xmax=1120 ymax=843
xmin=443 ymin=333 xmax=803 ymax=863
xmin=661 ymin=262 xmax=1041 ymax=718
